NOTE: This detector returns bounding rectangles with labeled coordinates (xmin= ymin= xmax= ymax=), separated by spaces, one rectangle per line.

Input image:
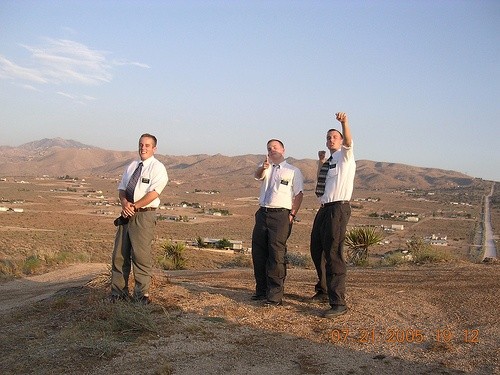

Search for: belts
xmin=133 ymin=207 xmax=156 ymax=212
xmin=259 ymin=207 xmax=288 ymax=212
xmin=321 ymin=201 xmax=349 ymax=208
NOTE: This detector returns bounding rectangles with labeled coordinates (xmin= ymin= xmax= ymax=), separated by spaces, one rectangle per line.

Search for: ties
xmin=125 ymin=162 xmax=143 ymax=204
xmin=263 ymin=165 xmax=280 ymax=205
xmin=315 ymin=155 xmax=333 ymax=198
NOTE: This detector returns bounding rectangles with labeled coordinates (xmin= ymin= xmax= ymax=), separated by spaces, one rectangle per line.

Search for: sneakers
xmin=322 ymin=307 xmax=347 ymax=316
xmin=309 ymin=292 xmax=330 ymax=303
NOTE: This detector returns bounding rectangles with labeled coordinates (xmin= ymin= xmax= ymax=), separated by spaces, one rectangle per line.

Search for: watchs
xmin=289 ymin=211 xmax=296 ymax=218
xmin=130 ymin=204 xmax=137 ymax=212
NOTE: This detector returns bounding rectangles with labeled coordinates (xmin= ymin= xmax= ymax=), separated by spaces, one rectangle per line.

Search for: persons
xmin=251 ymin=139 xmax=305 ymax=308
xmin=104 ymin=134 xmax=168 ymax=305
xmin=310 ymin=111 xmax=357 ymax=318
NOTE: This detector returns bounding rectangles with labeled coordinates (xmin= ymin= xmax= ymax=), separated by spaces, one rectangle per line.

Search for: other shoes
xmin=111 ymin=295 xmax=128 ymax=304
xmin=137 ymin=296 xmax=153 ymax=305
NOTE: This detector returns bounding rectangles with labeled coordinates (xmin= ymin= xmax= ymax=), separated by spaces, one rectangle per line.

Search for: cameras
xmin=114 ymin=216 xmax=129 ymax=226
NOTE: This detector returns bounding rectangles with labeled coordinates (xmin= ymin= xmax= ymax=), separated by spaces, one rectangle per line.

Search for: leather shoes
xmin=251 ymin=295 xmax=268 ymax=300
xmin=264 ymin=300 xmax=282 ymax=307
